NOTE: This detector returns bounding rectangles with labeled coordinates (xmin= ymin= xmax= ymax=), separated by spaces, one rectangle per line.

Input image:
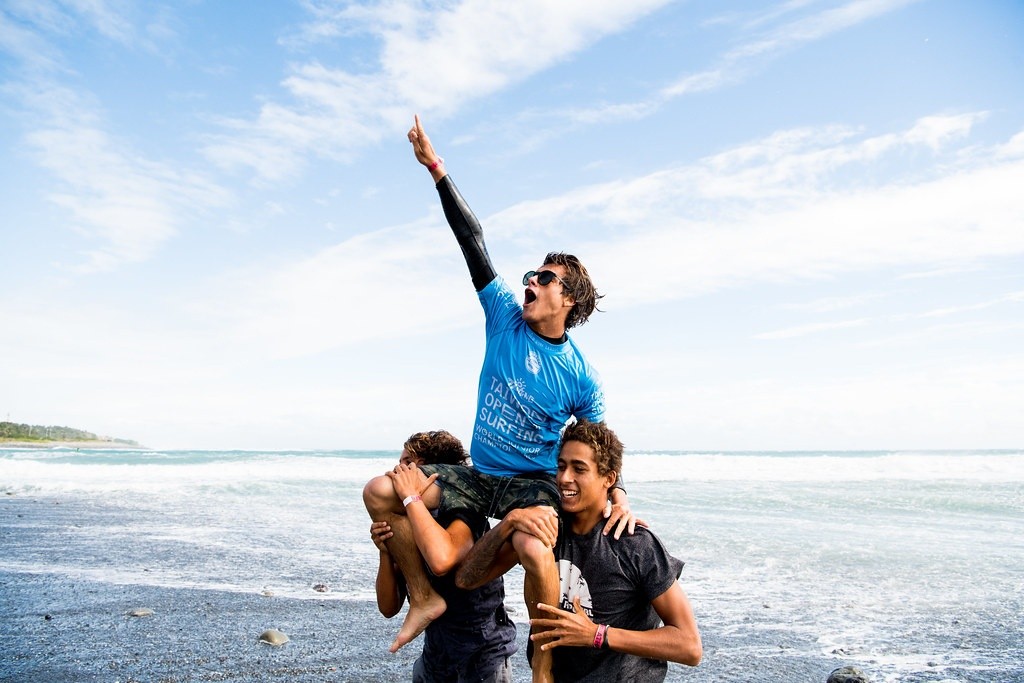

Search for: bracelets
xmin=593 ymin=623 xmax=610 ymax=650
xmin=403 ymin=496 xmax=422 ymax=507
xmin=427 ymin=156 xmax=444 ymax=173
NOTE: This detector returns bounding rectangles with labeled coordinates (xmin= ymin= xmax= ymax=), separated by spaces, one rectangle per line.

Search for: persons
xmin=363 ymin=115 xmax=650 ymax=683
xmin=456 ymin=418 xmax=703 ymax=683
xmin=370 ymin=430 xmax=520 ymax=683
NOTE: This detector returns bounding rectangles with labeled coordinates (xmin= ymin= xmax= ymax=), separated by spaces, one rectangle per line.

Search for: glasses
xmin=523 ymin=271 xmax=570 ymax=290
